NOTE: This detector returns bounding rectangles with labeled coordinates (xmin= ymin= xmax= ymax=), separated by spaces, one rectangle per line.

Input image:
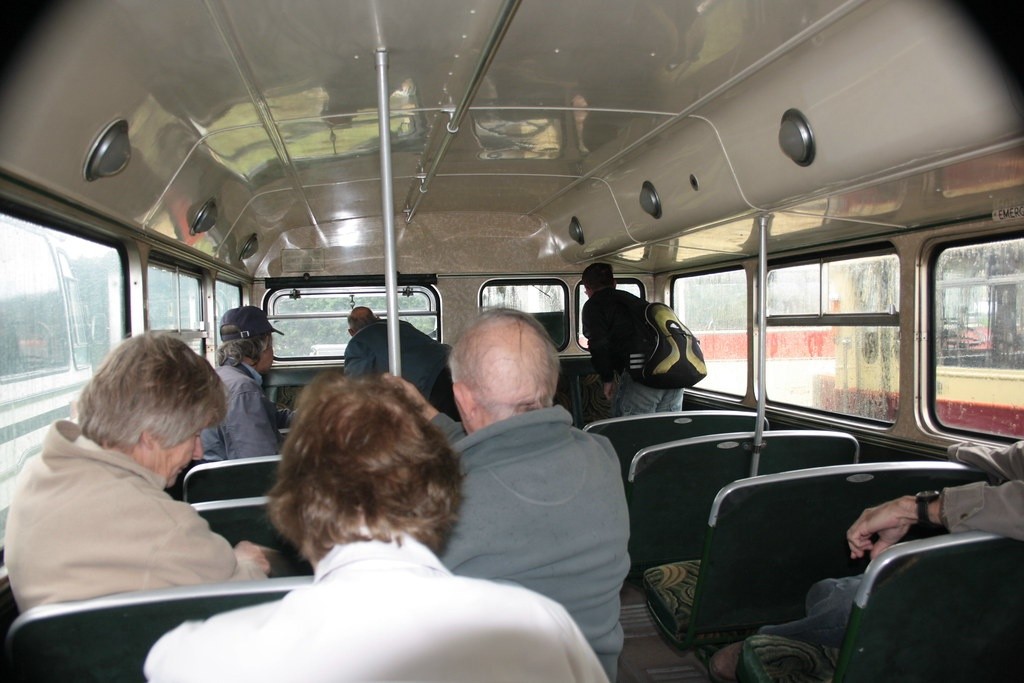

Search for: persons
xmin=582 ymin=262 xmax=707 ymax=417
xmin=382 ymin=307 xmax=633 ymax=683
xmin=342 ymin=307 xmax=462 ymax=419
xmin=143 ymin=374 xmax=609 ymax=683
xmin=708 ymin=438 xmax=1024 ymax=683
xmin=6 ymin=331 xmax=285 ymax=614
xmin=200 ymin=306 xmax=284 ymax=459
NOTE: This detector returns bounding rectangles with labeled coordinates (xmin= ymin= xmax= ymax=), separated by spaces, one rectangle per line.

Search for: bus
xmin=0 ymin=214 xmax=107 ymax=560
xmin=671 ymin=240 xmax=1024 ymax=443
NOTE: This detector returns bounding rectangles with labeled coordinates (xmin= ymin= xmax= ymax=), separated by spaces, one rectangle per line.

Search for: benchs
xmin=0 ymin=410 xmax=1024 ymax=683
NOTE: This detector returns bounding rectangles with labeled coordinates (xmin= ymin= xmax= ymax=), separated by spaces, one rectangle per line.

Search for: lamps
xmin=568 ymin=215 xmax=585 ymax=246
xmin=238 ymin=232 xmax=260 ymax=261
xmin=639 ymin=180 xmax=663 ymax=220
xmin=188 ymin=195 xmax=218 ymax=235
xmin=778 ymin=108 xmax=816 ymax=167
xmin=84 ymin=118 xmax=132 ymax=181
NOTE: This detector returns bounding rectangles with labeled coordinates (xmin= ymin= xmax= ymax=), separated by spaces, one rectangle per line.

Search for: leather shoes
xmin=710 ymin=640 xmax=744 ymax=683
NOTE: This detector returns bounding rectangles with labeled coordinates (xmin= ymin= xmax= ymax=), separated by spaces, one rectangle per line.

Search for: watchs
xmin=916 ymin=490 xmax=940 ymax=525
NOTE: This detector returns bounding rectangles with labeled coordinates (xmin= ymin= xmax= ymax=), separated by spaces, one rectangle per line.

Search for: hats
xmin=219 ymin=306 xmax=285 ymax=341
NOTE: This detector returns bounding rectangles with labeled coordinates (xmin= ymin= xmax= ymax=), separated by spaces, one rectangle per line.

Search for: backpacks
xmin=639 ymin=301 xmax=707 ymax=390
